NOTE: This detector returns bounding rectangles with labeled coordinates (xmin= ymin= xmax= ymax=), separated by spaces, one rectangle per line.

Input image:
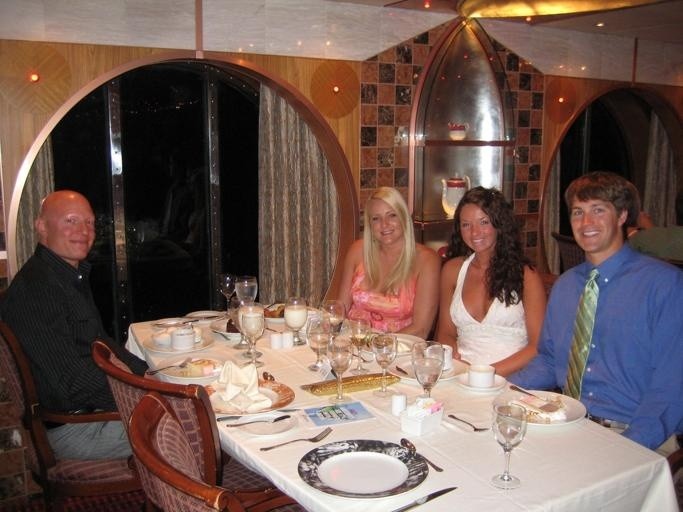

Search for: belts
xmin=42 ymin=410 xmax=111 ymax=430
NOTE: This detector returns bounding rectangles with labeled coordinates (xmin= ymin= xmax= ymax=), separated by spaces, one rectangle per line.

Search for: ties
xmin=563 ymin=267 xmax=601 ymax=399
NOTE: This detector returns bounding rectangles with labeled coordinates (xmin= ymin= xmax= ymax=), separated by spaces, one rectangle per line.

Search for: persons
xmin=430 ymin=186 xmax=547 ymax=377
xmin=333 ymin=187 xmax=440 ymax=340
xmin=3 ymin=190 xmax=155 ymax=465
xmin=505 ymin=173 xmax=683 ymax=459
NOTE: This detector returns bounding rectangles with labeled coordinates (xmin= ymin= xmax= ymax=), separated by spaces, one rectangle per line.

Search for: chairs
xmin=0 ymin=325 xmax=149 ymax=511
xmin=126 ymin=391 xmax=246 ymax=512
xmin=91 ymin=336 xmax=278 ymax=498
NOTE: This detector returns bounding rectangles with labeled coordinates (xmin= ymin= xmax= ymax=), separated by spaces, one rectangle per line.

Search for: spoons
xmin=448 ymin=414 xmax=490 ymax=433
xmin=224 ymin=414 xmax=292 ymax=429
xmin=400 ymin=438 xmax=444 ymax=473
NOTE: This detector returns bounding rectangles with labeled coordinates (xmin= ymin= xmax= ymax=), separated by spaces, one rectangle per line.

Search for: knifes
xmin=510 ymin=384 xmax=536 ymax=396
xmin=391 ymin=486 xmax=458 ymax=512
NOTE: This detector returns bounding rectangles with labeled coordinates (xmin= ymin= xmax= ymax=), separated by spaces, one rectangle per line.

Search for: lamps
xmin=456 ymin=0 xmax=644 ymax=17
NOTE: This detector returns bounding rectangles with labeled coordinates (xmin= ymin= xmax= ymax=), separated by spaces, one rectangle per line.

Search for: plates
xmin=201 ymin=379 xmax=294 ymax=418
xmin=260 ymin=302 xmax=286 ymax=322
xmin=156 ymin=352 xmax=224 ymax=381
xmin=183 ymin=310 xmax=223 ymax=329
xmin=369 ymin=330 xmax=427 ymax=357
xmin=493 ymin=390 xmax=588 ymax=428
xmin=238 ymin=410 xmax=299 ymax=437
xmin=455 ymin=371 xmax=508 ymax=392
xmin=151 ymin=316 xmax=192 ymax=329
xmin=142 ymin=324 xmax=216 ymax=354
xmin=387 ymin=351 xmax=470 ymax=382
xmin=296 ymin=436 xmax=431 ymax=501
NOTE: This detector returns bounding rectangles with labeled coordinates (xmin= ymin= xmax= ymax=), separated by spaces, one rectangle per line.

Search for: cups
xmin=447 ymin=121 xmax=468 ymax=142
xmin=468 ymin=363 xmax=495 ymax=388
xmin=426 ymin=345 xmax=453 ymax=371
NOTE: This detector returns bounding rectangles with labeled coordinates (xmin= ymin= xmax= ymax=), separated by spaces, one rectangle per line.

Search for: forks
xmin=258 ymin=425 xmax=333 ymax=454
xmin=145 ymin=355 xmax=194 ymax=376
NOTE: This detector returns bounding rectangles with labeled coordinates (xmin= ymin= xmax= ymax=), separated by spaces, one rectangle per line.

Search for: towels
xmin=207 ymin=361 xmax=272 ymax=412
xmin=402 ymin=399 xmax=445 ymax=434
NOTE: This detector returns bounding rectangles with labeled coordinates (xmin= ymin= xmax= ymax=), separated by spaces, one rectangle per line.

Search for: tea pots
xmin=441 ymin=172 xmax=470 ymax=219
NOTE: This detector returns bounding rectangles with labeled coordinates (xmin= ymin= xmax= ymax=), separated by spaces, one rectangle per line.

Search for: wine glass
xmin=283 ymin=297 xmax=308 ymax=347
xmin=349 ymin=311 xmax=372 ymax=377
xmin=306 ymin=317 xmax=329 ymax=372
xmin=324 ymin=299 xmax=346 ymax=337
xmin=373 ymin=334 xmax=398 ymax=398
xmin=234 ymin=276 xmax=258 ymax=304
xmin=215 ymin=273 xmax=237 ymax=317
xmin=241 ymin=312 xmax=265 ymax=369
xmin=227 ymin=296 xmax=254 ymax=349
xmin=490 ymin=401 xmax=528 ymax=491
xmin=237 ymin=302 xmax=263 ymax=359
xmin=411 ymin=340 xmax=445 ymax=401
xmin=324 ymin=335 xmax=354 ymax=405
